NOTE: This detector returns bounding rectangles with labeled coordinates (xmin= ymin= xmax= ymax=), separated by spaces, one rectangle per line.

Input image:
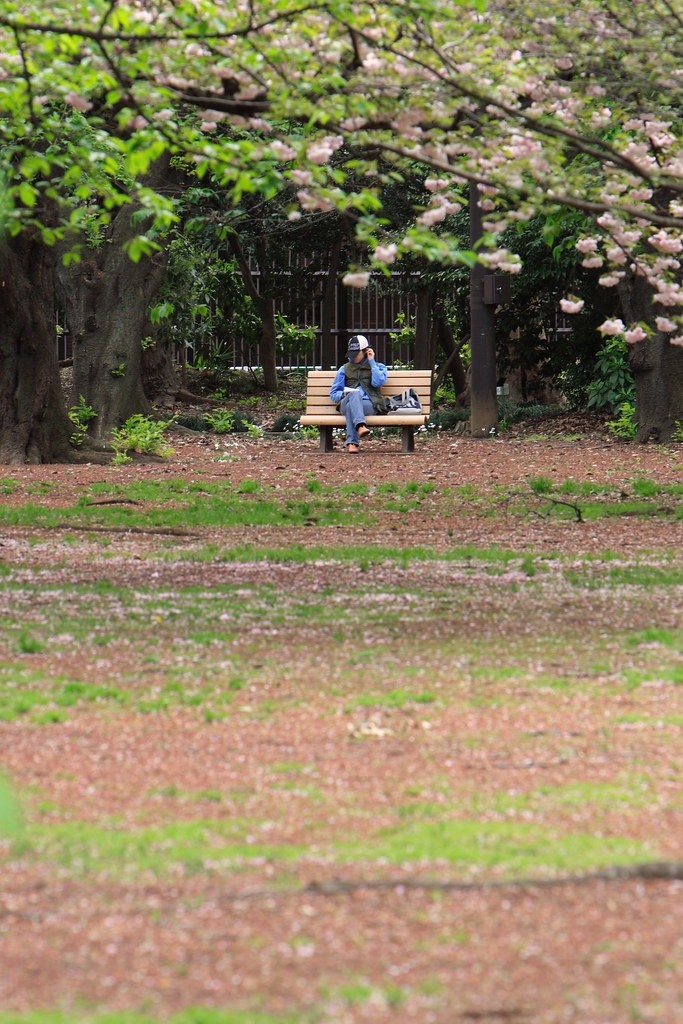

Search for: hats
xmin=345 ymin=335 xmax=369 ymax=358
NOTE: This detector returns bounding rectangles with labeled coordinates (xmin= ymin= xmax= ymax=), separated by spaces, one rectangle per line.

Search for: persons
xmin=329 ymin=335 xmax=388 ymax=453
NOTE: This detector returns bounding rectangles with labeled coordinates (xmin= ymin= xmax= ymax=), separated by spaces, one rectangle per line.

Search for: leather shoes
xmin=349 ymin=444 xmax=359 ymax=453
xmin=359 ymin=426 xmax=370 ymax=437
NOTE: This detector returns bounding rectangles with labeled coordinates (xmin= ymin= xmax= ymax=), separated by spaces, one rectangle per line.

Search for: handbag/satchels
xmin=383 ymin=388 xmax=422 ymax=414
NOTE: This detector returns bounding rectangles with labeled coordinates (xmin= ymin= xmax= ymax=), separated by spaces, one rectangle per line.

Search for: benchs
xmin=300 ymin=369 xmax=432 ymax=454
